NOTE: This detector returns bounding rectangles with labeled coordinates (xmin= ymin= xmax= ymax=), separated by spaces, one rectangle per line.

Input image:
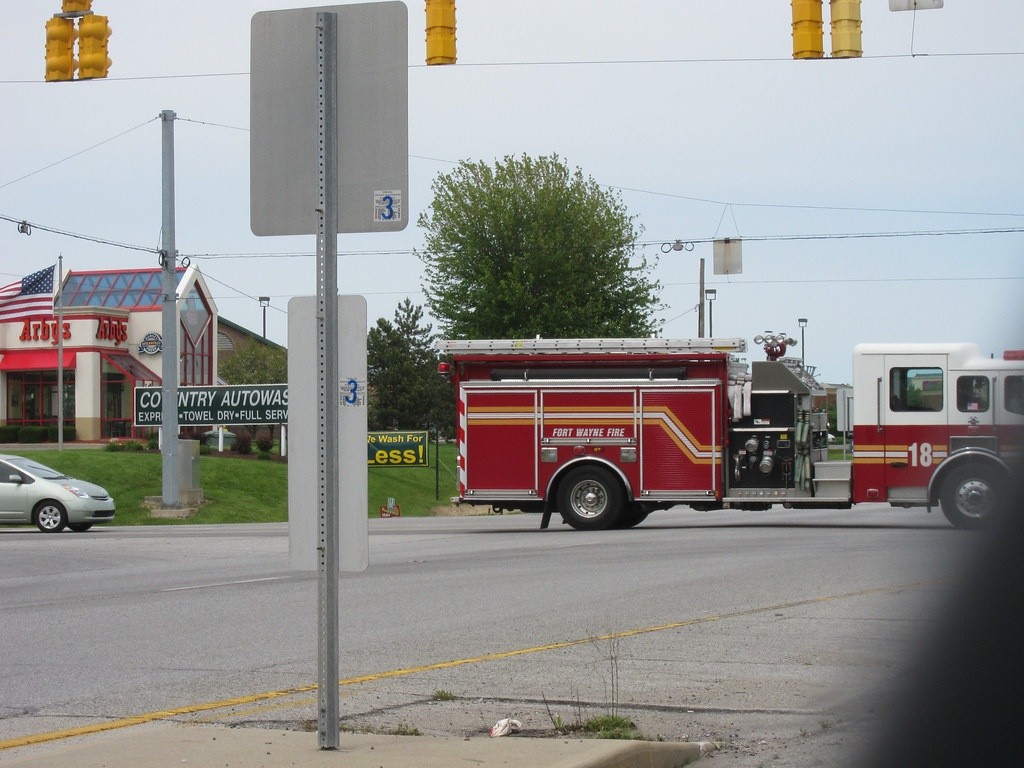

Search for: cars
xmin=0 ymin=454 xmax=117 ymax=532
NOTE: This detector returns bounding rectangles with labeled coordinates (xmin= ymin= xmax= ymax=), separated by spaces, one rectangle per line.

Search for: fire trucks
xmin=432 ymin=332 xmax=1024 ymax=532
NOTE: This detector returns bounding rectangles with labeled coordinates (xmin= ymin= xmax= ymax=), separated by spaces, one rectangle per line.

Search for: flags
xmin=0 ymin=263 xmax=57 ymax=323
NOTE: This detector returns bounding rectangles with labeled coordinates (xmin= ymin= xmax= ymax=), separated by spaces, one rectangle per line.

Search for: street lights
xmin=705 ymin=287 xmax=717 ymax=339
xmin=258 ymin=295 xmax=271 ymax=337
xmin=798 ymin=316 xmax=809 ymax=366
